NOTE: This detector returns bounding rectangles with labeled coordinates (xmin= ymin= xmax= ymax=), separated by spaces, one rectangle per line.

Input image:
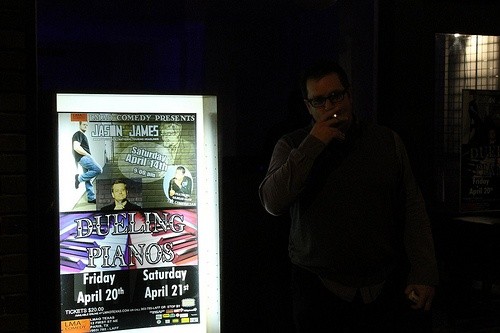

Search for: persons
xmin=258 ymin=60 xmax=440 ymax=332
xmin=72 ymin=120 xmax=193 ymax=213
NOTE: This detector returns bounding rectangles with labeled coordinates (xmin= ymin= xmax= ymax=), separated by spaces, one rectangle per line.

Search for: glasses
xmin=306 ymin=89 xmax=346 ymax=109
